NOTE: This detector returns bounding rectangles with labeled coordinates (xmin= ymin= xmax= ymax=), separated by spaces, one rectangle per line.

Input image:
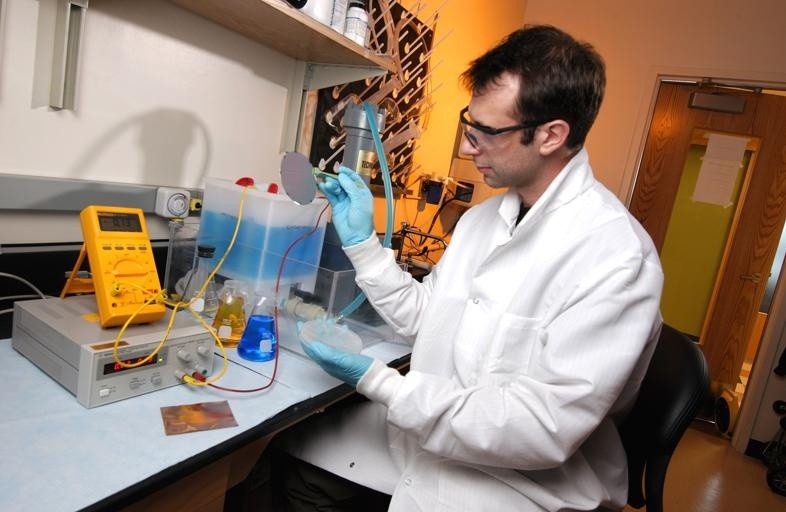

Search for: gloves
xmin=295 ymin=319 xmax=374 ymax=390
xmin=318 ymin=165 xmax=375 ymax=248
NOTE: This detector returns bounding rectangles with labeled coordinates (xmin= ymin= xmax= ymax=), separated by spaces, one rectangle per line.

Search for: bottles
xmin=211 ymin=279 xmax=247 ymax=348
xmin=332 ymin=0 xmax=347 ymax=35
xmin=179 ymin=244 xmax=219 ymax=318
xmin=237 ymin=290 xmax=277 ymax=362
xmin=345 ymin=1 xmax=368 ymax=46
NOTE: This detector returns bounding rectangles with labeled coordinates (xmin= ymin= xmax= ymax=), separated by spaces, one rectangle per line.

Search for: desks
xmin=0 ymin=311 xmax=412 ymax=512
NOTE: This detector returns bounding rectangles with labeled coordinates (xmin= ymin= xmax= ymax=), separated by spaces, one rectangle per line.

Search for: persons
xmin=225 ymin=23 xmax=666 ymax=511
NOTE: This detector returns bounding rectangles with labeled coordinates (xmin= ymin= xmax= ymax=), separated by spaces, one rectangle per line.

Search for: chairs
xmin=609 ymin=323 xmax=708 ymax=512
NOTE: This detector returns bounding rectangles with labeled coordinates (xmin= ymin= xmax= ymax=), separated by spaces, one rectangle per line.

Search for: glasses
xmin=460 ymin=107 xmax=549 ymax=151
